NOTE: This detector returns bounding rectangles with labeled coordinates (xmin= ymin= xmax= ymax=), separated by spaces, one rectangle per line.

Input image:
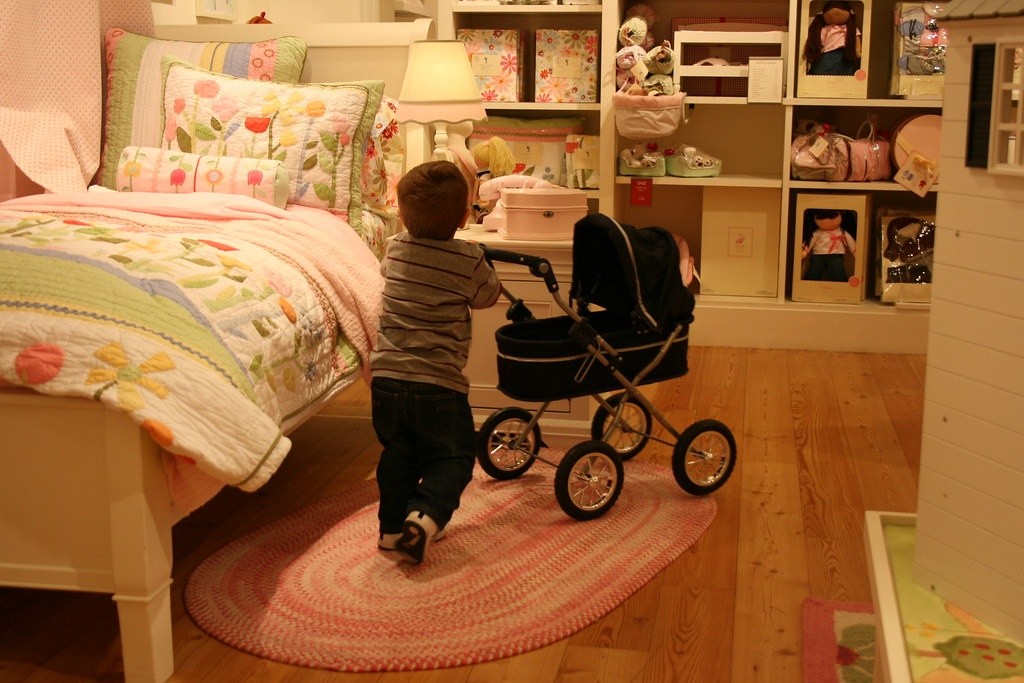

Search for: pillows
xmin=96 ymin=28 xmax=308 ymax=192
xmin=156 ymin=54 xmax=386 ymax=237
xmin=357 ymin=95 xmax=399 ymax=219
xmin=115 ymin=146 xmax=289 ymax=210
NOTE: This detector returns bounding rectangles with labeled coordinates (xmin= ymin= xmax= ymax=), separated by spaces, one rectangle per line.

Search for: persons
xmin=368 ymin=159 xmax=503 ymax=564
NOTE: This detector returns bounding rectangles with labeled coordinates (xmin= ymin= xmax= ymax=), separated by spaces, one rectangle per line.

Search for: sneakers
xmin=377 ymin=527 xmax=447 ymax=549
xmin=394 ymin=512 xmax=437 ymax=564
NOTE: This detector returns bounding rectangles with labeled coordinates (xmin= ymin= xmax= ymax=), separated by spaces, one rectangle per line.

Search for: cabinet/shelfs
xmin=437 ymin=1 xmax=946 ymax=353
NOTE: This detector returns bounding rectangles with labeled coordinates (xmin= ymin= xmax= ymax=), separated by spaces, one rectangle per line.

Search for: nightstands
xmin=451 ymin=225 xmax=603 ymax=437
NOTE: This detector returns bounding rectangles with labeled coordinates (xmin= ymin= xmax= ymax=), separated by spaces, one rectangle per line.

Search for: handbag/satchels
xmin=830 ymin=116 xmax=895 ymax=182
xmin=789 ymin=118 xmax=855 ymax=181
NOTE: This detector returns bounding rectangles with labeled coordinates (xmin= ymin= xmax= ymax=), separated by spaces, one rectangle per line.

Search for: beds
xmin=0 ymin=18 xmax=439 ymax=683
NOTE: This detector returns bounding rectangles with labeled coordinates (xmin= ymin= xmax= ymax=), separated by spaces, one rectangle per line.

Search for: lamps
xmin=396 ymin=40 xmax=488 ymax=230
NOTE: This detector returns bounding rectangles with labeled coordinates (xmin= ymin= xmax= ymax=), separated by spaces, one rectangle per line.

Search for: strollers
xmin=475 ymin=211 xmax=739 ymax=521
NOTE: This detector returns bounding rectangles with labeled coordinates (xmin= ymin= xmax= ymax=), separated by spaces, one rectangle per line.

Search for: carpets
xmin=184 ymin=448 xmax=717 ymax=672
xmin=797 ymin=594 xmax=878 ymax=683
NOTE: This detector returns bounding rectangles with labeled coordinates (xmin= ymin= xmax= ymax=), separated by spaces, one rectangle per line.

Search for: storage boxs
xmin=496 ymin=189 xmax=589 ymax=241
